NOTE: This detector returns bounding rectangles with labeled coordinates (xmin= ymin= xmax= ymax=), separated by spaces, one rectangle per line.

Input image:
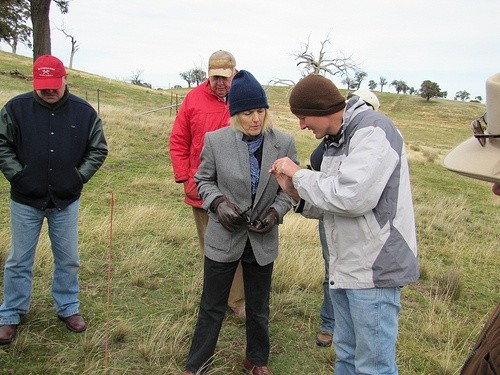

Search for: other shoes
xmin=242 ymin=358 xmax=274 ymax=375
xmin=315 ymin=329 xmax=333 ymax=347
xmin=234 ymin=306 xmax=246 ymax=319
xmin=181 ymin=369 xmax=199 ymax=375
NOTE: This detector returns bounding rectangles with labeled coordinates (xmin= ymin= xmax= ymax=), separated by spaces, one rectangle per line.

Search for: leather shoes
xmin=58 ymin=313 xmax=87 ymax=332
xmin=0 ymin=323 xmax=18 ymax=345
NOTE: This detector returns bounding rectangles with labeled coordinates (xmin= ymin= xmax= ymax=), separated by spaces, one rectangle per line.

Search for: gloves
xmin=247 ymin=208 xmax=279 ymax=234
xmin=210 ymin=196 xmax=249 ymax=233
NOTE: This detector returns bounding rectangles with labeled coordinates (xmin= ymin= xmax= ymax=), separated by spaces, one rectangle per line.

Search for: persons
xmin=178 ymin=70 xmax=300 ymax=375
xmin=440 ymin=72 xmax=500 ymax=375
xmin=310 ymin=88 xmax=381 ymax=347
xmin=268 ymin=73 xmax=421 ymax=375
xmin=169 ymin=49 xmax=245 ymax=321
xmin=0 ymin=54 xmax=108 ymax=344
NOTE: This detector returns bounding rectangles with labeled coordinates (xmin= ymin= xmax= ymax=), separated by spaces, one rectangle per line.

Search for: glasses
xmin=471 ymin=112 xmax=500 ymax=147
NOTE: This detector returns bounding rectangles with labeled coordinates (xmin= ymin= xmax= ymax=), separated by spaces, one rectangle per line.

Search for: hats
xmin=288 ymin=73 xmax=347 ymax=116
xmin=208 ymin=49 xmax=237 ymax=78
xmin=348 ymin=87 xmax=380 ymax=112
xmin=32 ymin=55 xmax=69 ymax=90
xmin=228 ymin=70 xmax=270 ymax=116
xmin=440 ymin=71 xmax=500 ymax=183
